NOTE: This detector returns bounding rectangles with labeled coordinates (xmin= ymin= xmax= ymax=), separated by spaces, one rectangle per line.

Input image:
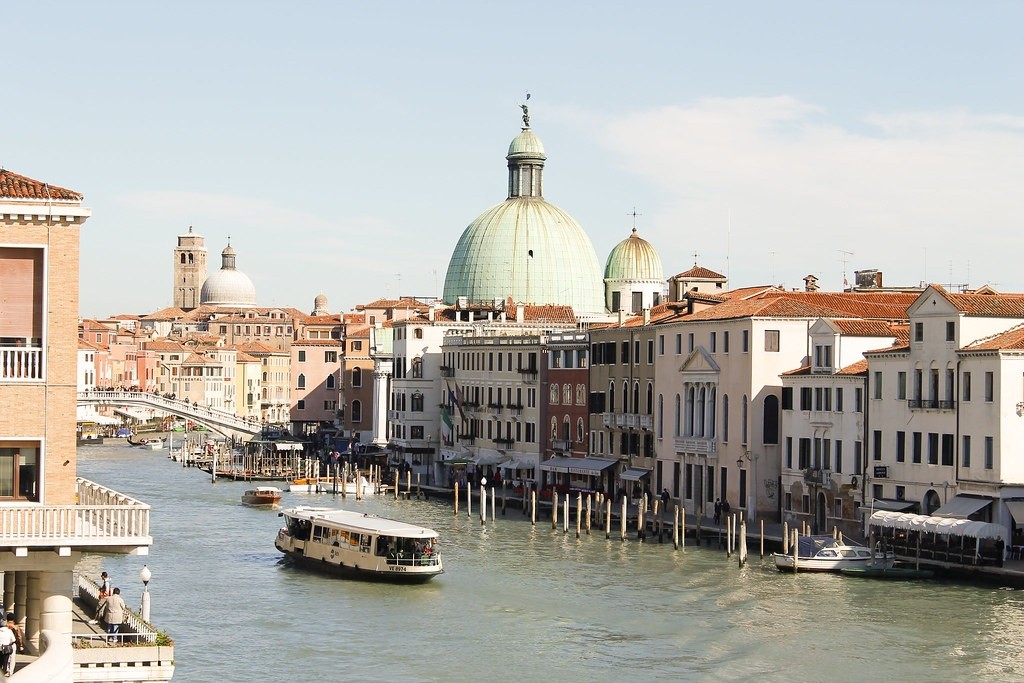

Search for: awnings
xmin=930 ymin=495 xmax=994 ymax=519
xmin=540 ymin=456 xmax=616 ymax=476
xmin=863 ymin=498 xmax=916 ymax=512
xmin=1005 ymin=498 xmax=1024 ymax=529
xmin=620 ymin=468 xmax=648 ymax=482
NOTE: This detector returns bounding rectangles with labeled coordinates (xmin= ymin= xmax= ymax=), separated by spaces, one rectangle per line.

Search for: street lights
xmin=426 ymin=435 xmax=431 ymax=486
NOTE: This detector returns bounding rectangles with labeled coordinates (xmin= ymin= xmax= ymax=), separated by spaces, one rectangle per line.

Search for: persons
xmin=644 ymin=489 xmax=653 ymax=510
xmin=633 ymin=485 xmax=641 ymax=498
xmin=291 ymin=519 xmax=311 ymax=540
xmin=661 ymin=488 xmax=670 ymax=512
xmin=0 ymin=612 xmax=24 ymax=677
xmin=715 ymin=498 xmax=730 ymax=525
xmin=388 ymin=538 xmax=437 ymax=565
xmin=85 ymin=385 xmax=198 ymax=411
xmin=89 ymin=572 xmax=125 ymax=642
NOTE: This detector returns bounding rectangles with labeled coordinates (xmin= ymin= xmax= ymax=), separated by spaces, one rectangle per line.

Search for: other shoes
xmin=5 ymin=673 xmax=10 ymax=676
xmin=109 ymin=638 xmax=118 ymax=642
xmin=89 ymin=619 xmax=99 ymax=624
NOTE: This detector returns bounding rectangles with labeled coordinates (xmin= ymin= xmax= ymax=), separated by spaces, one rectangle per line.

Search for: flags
xmin=456 ymin=385 xmax=464 ymax=407
xmin=441 ymin=408 xmax=453 ymax=438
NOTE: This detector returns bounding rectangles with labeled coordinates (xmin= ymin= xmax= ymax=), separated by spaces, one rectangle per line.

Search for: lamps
xmin=736 ymin=451 xmax=752 ymax=469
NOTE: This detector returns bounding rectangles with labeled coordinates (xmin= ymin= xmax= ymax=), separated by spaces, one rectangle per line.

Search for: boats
xmin=275 ymin=506 xmax=445 ymax=586
xmin=773 ymin=546 xmax=896 ymax=573
xmin=241 ymin=487 xmax=282 ymax=508
xmin=320 ymin=476 xmax=388 ymax=495
xmin=112 ymin=433 xmax=133 ymax=438
xmin=140 ymin=439 xmax=164 ymax=451
xmin=127 ymin=434 xmax=168 ymax=445
xmin=196 ymin=426 xmax=210 ymax=431
xmin=841 ymin=567 xmax=935 ymax=579
xmin=174 ymin=428 xmax=184 ymax=432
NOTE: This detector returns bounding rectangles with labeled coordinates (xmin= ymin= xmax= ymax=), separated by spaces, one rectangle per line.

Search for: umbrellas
xmin=497 ymin=459 xmax=533 ymax=470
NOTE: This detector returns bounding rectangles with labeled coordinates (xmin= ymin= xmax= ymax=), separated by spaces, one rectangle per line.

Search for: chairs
xmin=1013 ymin=537 xmax=1024 ymax=560
xmin=875 ymin=541 xmax=881 ymax=553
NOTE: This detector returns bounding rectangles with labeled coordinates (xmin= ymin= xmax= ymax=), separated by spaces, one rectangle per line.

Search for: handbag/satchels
xmin=99 ymin=588 xmax=111 ymax=597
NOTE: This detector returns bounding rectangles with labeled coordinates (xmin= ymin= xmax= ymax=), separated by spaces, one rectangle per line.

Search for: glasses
xmin=101 ymin=575 xmax=102 ymax=577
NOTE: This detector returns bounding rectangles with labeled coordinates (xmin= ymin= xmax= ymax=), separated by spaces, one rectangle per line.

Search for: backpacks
xmin=2 ymin=641 xmax=15 ymax=655
